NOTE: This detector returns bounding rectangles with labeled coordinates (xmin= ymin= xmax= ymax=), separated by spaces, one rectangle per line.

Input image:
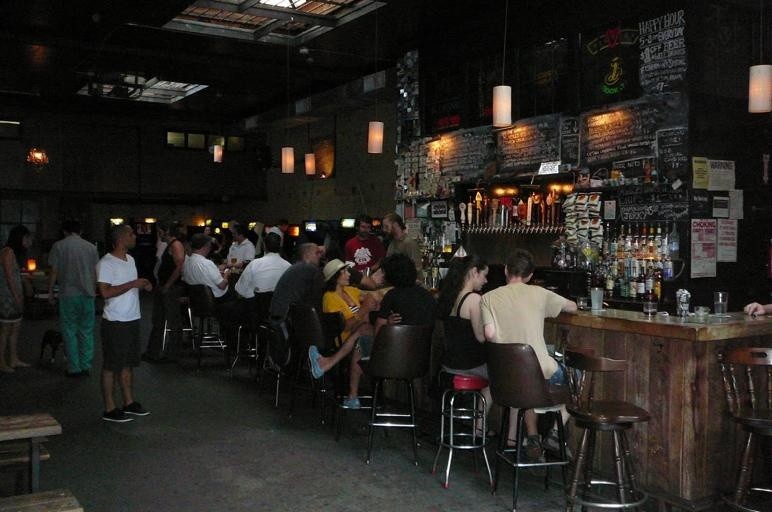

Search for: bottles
xmin=599 ymin=216 xmax=680 ymax=302
xmin=590 ymin=161 xmax=658 ymax=188
xmin=418 ymin=233 xmax=454 ymax=291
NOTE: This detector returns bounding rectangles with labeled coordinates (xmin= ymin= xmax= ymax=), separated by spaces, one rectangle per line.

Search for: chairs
xmin=718 ymin=347 xmax=772 ymax=512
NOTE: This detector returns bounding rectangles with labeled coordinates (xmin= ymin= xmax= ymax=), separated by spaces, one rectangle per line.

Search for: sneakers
xmin=123 ymin=401 xmax=150 ymax=416
xmin=541 ymin=429 xmax=574 ymax=460
xmin=342 ymin=399 xmax=361 ymax=409
xmin=308 ymin=344 xmax=326 ymax=379
xmin=102 ymin=408 xmax=134 ymax=422
xmin=524 ymin=435 xmax=548 ymax=478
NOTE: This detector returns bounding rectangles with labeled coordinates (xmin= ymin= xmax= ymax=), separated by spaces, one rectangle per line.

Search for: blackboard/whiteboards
xmin=636 ymin=6 xmax=687 ymax=95
xmin=441 ymin=91 xmax=691 ymax=183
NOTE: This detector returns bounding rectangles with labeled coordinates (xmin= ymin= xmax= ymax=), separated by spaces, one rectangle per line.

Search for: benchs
xmin=0 ymin=488 xmax=83 ymax=512
xmin=0 ymin=444 xmax=51 ymax=466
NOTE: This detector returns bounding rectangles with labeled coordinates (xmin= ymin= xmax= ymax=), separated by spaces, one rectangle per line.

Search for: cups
xmin=675 ymin=288 xmax=691 ymax=317
xmin=641 ymin=301 xmax=659 ymax=316
xmin=361 ymin=266 xmax=372 ymax=279
xmin=714 ymin=292 xmax=728 ymax=317
xmin=219 ymin=257 xmax=250 ymax=274
xmin=575 ymin=296 xmax=587 ymax=310
xmin=590 ymin=287 xmax=604 ymax=310
xmin=694 ymin=304 xmax=711 ymax=323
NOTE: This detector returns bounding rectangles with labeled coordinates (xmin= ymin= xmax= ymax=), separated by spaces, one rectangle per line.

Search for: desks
xmin=0 ymin=410 xmax=63 ymax=492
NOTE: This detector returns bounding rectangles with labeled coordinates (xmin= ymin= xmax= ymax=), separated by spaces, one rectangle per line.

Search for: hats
xmin=323 ymin=258 xmax=350 ymax=283
xmin=192 ymin=233 xmax=211 ymax=248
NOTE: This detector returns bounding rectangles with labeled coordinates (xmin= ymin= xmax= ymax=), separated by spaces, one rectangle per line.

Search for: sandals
xmin=506 ymin=437 xmax=518 ymax=449
xmin=475 ymin=428 xmax=496 ymax=438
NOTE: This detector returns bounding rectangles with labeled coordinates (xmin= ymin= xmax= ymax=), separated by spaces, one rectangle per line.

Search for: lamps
xmin=304 ymin=124 xmax=316 ymax=177
xmin=213 ymin=144 xmax=224 ymax=164
xmin=367 ymin=0 xmax=384 ymax=154
xmin=280 ymin=29 xmax=295 ymax=174
xmin=747 ymin=0 xmax=772 ymax=114
xmin=492 ymin=0 xmax=512 ymax=128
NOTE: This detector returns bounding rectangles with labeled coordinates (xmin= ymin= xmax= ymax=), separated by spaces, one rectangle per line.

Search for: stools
xmin=310 ymin=308 xmax=392 ymax=442
xmin=355 ymin=321 xmax=433 ymax=465
xmin=482 ymin=339 xmax=575 ymax=511
xmin=158 ymin=283 xmax=304 ymax=409
xmin=561 ymin=345 xmax=652 ymax=512
xmin=432 ymin=372 xmax=498 ymax=497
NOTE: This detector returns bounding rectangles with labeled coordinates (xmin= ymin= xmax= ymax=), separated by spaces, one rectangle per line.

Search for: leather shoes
xmin=140 ymin=352 xmax=155 ymax=362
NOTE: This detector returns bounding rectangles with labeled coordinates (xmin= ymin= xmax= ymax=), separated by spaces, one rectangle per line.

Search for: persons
xmin=743 ymin=301 xmax=772 ymax=318
xmin=308 ymin=253 xmax=437 ymax=411
xmin=438 ymin=254 xmax=527 ymax=448
xmin=47 ymin=220 xmax=100 ymax=379
xmin=0 ymin=225 xmax=34 ymax=374
xmin=479 ymin=248 xmax=579 ymax=478
xmin=142 ymin=213 xmax=426 ymax=366
xmin=95 ymin=225 xmax=151 ymax=423
